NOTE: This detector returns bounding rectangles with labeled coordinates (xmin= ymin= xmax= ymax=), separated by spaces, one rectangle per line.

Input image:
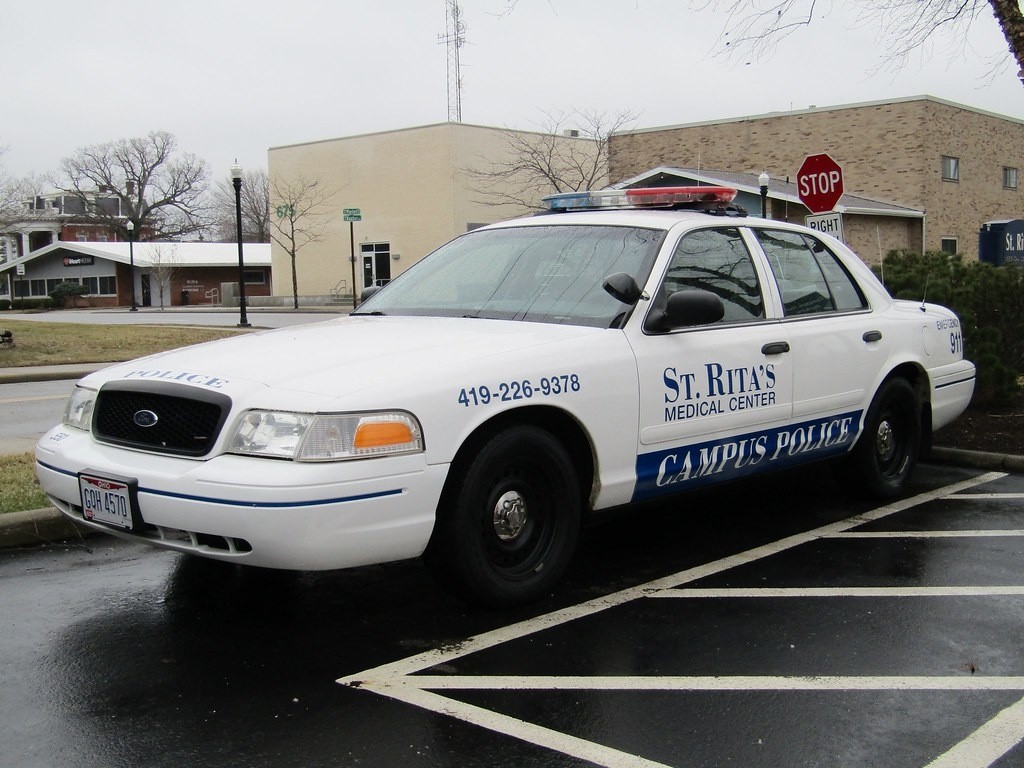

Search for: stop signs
xmin=797 ymin=154 xmax=844 ymax=215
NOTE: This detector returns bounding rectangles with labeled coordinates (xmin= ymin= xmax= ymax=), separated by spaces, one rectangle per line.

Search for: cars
xmin=34 ymin=186 xmax=977 ymax=611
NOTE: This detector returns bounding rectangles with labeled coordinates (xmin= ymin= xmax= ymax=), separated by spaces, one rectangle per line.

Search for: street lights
xmin=127 ymin=219 xmax=139 ymax=311
xmin=758 ymin=169 xmax=769 ymax=218
xmin=230 ymin=158 xmax=252 ymax=327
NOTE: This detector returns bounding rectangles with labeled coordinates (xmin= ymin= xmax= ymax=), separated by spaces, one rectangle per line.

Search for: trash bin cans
xmin=181 ymin=291 xmax=189 ymax=305
